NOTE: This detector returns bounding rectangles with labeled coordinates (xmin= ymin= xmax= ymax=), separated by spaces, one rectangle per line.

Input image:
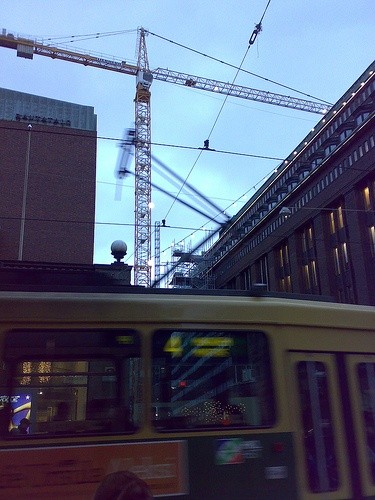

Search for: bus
xmin=0 ymin=287 xmax=375 ymax=500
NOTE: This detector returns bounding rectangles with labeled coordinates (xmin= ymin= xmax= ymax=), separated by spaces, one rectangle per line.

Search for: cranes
xmin=1 ymin=23 xmax=336 ymax=287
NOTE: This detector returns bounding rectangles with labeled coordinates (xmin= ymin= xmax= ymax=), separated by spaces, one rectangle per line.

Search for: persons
xmin=9 ymin=418 xmax=32 ymax=435
